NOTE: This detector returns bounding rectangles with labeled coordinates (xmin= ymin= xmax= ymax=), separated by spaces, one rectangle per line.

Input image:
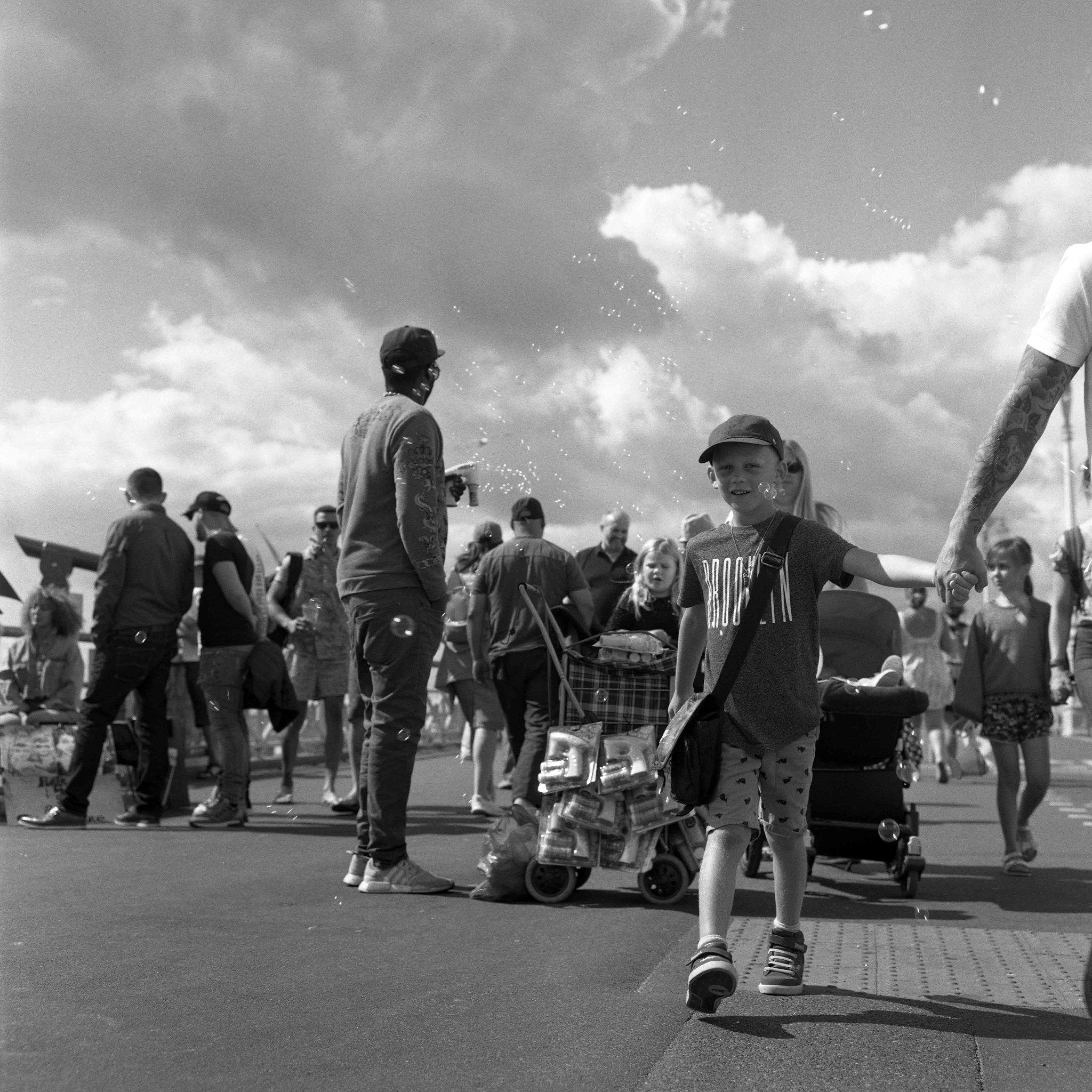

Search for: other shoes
xmin=18 ymin=805 xmax=88 ymax=829
xmin=114 ymin=805 xmax=157 ymax=827
xmin=497 ymin=773 xmax=512 ymax=788
xmin=512 ymin=798 xmax=538 ymax=832
xmin=758 ymin=927 xmax=808 ymax=995
xmin=332 ymin=799 xmax=358 ymax=811
xmin=470 ymin=794 xmax=508 ymax=816
xmin=189 ymin=786 xmax=247 ymax=828
xmin=858 ymin=654 xmax=903 ymax=687
xmin=935 ymin=759 xmax=948 ymax=784
xmin=684 ymin=940 xmax=738 ymax=1014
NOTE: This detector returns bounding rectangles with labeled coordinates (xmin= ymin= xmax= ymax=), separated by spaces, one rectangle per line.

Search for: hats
xmin=512 ymin=497 xmax=543 ymax=520
xmin=182 ymin=491 xmax=232 ymax=521
xmin=698 ymin=414 xmax=784 ymax=465
xmin=473 ymin=521 xmax=504 ymax=544
xmin=677 ymin=513 xmax=716 ymax=542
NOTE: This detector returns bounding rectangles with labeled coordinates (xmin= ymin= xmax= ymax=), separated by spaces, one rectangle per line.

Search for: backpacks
xmin=442 ymin=568 xmax=491 ymax=646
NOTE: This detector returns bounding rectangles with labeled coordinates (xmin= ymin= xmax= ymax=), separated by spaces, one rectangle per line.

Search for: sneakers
xmin=343 ymin=850 xmax=454 ymax=893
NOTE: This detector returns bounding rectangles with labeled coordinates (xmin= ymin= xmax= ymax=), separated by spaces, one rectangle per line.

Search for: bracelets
xmin=1048 ymin=658 xmax=1066 ymax=667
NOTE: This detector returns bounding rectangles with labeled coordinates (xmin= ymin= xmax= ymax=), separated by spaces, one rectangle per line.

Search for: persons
xmin=336 ymin=324 xmax=467 ymax=895
xmin=0 ymin=240 xmax=1092 ymax=1017
xmin=667 ymin=416 xmax=980 ymax=1013
xmin=16 ymin=468 xmax=196 ymax=832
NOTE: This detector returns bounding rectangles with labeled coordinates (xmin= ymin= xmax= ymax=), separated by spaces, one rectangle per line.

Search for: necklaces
xmin=731 ymin=508 xmax=777 ymax=588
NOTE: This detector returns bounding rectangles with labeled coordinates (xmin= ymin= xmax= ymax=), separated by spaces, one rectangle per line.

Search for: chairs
xmin=23 ymin=650 xmax=98 ymax=811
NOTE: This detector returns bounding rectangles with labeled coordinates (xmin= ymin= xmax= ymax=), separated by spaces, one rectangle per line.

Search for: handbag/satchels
xmin=266 ymin=551 xmax=304 ymax=646
xmin=653 ymin=692 xmax=723 ymax=817
xmin=954 ymin=720 xmax=989 ymax=778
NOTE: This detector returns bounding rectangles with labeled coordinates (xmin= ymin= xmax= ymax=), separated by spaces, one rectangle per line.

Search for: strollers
xmin=515 ymin=580 xmax=706 ymax=906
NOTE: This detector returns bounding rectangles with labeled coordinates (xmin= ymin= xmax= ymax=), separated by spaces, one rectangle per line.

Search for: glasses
xmin=315 ymin=522 xmax=340 ymax=529
xmin=787 ymin=461 xmax=801 ymax=473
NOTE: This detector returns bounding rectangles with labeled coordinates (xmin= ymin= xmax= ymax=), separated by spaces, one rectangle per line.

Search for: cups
xmin=302 ymin=604 xmax=319 ymax=626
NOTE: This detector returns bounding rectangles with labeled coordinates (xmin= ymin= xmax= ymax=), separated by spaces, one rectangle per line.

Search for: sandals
xmin=1001 ymin=823 xmax=1037 ymax=875
xmin=197 ymin=762 xmax=223 ymax=781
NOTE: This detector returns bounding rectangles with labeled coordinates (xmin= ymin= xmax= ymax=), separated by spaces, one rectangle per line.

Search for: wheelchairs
xmin=745 ymin=588 xmax=931 ymax=897
